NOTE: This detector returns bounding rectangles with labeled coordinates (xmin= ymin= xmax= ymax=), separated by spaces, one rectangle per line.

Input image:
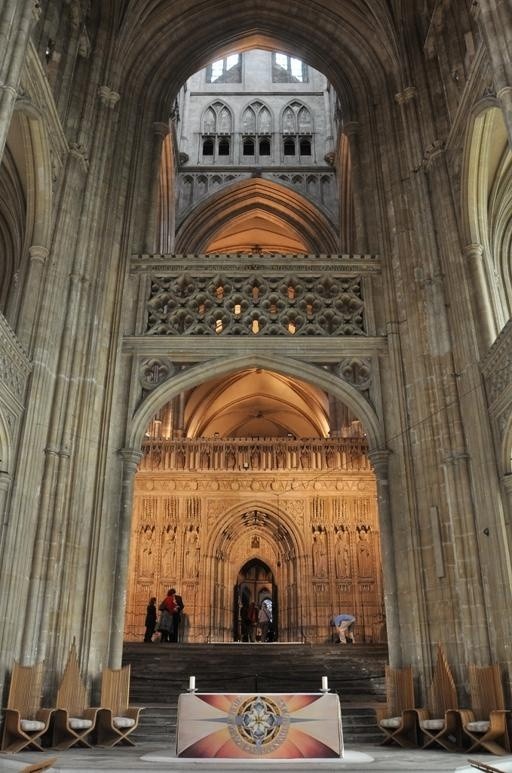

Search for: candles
xmin=189 ymin=675 xmax=196 ymax=688
xmin=321 ymin=675 xmax=328 ymax=689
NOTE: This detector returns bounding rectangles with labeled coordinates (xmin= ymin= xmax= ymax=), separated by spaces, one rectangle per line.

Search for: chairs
xmin=0 ymin=658 xmax=56 ymax=753
xmin=369 ymin=662 xmax=415 ymax=748
xmin=56 ymin=636 xmax=104 ymax=751
xmin=455 ymin=663 xmax=511 ymax=756
xmin=100 ymin=663 xmax=147 ymax=751
xmin=410 ymin=640 xmax=458 ymax=752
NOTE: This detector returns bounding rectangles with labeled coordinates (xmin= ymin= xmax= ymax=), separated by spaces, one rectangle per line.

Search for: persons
xmin=329 ymin=613 xmax=356 ymax=644
xmin=240 ymin=600 xmax=274 ymax=642
xmin=154 ymin=588 xmax=181 ymax=643
xmin=168 ymin=595 xmax=184 ymax=642
xmin=143 ymin=596 xmax=157 ymax=643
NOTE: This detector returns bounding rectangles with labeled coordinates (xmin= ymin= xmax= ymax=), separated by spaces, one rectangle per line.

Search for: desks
xmin=175 ymin=692 xmax=344 ymax=758
